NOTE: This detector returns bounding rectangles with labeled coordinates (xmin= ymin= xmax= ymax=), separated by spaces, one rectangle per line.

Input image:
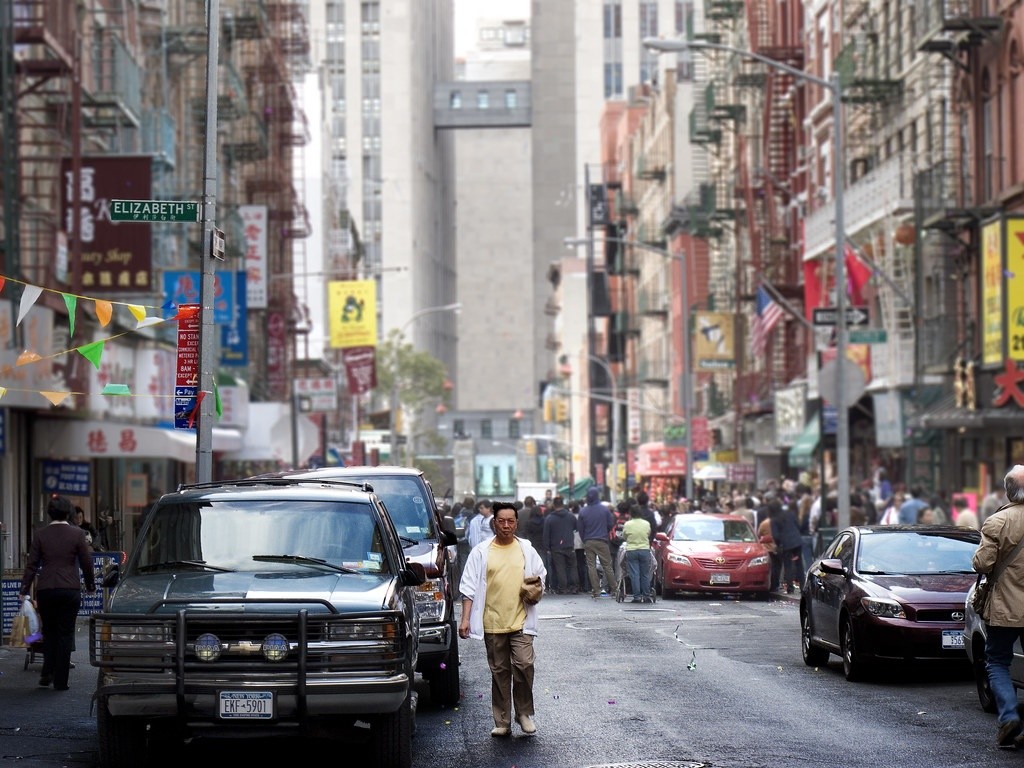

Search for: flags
xmin=844 ymin=245 xmax=875 ymax=305
xmin=750 ymin=286 xmax=784 ymax=359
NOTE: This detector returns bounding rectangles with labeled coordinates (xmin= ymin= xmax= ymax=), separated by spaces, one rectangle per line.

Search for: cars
xmin=956 ymin=572 xmax=1023 ymax=715
xmin=797 ymin=522 xmax=994 ymax=680
xmin=652 ymin=514 xmax=773 ymax=599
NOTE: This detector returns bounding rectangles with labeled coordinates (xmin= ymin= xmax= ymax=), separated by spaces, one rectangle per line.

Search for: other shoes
xmin=39 ymin=673 xmax=53 ymax=687
xmin=54 ymin=684 xmax=70 ymax=690
xmin=787 ymin=586 xmax=794 ymax=594
xmin=643 ymin=598 xmax=652 ymax=603
xmin=631 ymin=599 xmax=641 ymax=603
xmin=69 ymin=661 xmax=76 ymax=668
xmin=998 ymin=721 xmax=1022 ymax=746
xmin=515 ymin=715 xmax=536 ymax=733
xmin=491 ymin=726 xmax=512 ymax=737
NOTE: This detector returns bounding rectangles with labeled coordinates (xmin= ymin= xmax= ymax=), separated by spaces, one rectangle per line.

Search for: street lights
xmin=564 ymin=234 xmax=697 ymax=510
xmin=642 ymin=34 xmax=852 ymax=531
xmin=390 ymin=301 xmax=472 ymax=466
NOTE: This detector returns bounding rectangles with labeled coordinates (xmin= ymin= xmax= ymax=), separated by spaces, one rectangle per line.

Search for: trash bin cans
xmin=817 ymin=528 xmax=838 ymax=556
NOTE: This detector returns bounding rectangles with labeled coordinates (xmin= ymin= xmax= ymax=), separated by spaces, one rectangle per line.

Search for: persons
xmin=20 ymin=496 xmax=97 ymax=690
xmin=67 ymin=506 xmax=98 ymax=552
xmin=972 ymin=465 xmax=1024 ymax=752
xmin=767 ymin=499 xmax=806 ymax=594
xmin=577 ymin=487 xmax=618 ymax=599
xmin=981 ymin=479 xmax=1006 ymax=521
xmin=622 ymin=505 xmax=653 ymax=603
xmin=436 ymin=467 xmax=951 ymax=600
xmin=954 ymin=497 xmax=978 ymax=530
xmin=458 ymin=503 xmax=547 ymax=737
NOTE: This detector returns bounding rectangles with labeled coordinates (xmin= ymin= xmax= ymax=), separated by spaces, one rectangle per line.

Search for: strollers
xmin=614 ymin=541 xmax=657 ymax=604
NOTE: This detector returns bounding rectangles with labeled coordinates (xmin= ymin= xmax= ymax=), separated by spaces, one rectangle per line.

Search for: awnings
xmin=788 ymin=411 xmax=820 ymax=466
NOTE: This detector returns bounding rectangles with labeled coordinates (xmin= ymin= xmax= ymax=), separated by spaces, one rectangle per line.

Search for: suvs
xmin=87 ymin=474 xmax=425 ymax=768
xmin=239 ymin=464 xmax=464 ymax=710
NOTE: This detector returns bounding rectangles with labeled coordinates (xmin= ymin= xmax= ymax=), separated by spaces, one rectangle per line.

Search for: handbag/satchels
xmin=9 ymin=594 xmax=42 ymax=650
xmin=973 ymin=582 xmax=989 ymax=614
